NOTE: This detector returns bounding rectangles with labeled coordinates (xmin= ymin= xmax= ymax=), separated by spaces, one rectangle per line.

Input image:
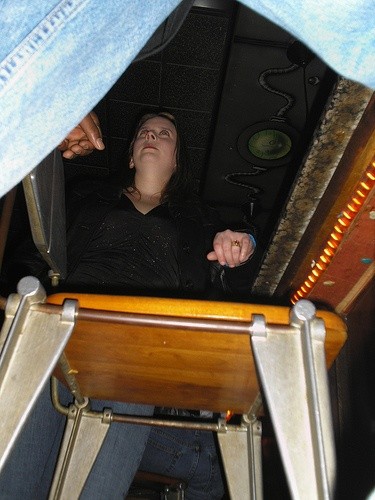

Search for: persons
xmin=1 ymin=1 xmax=374 ymax=197
xmin=0 ymin=109 xmax=264 ymax=500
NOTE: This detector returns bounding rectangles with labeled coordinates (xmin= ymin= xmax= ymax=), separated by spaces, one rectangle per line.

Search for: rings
xmin=231 ymin=239 xmax=241 ymax=247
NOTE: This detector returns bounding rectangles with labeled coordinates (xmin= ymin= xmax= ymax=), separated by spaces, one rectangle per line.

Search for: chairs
xmin=0 ymin=146 xmax=349 ymax=500
